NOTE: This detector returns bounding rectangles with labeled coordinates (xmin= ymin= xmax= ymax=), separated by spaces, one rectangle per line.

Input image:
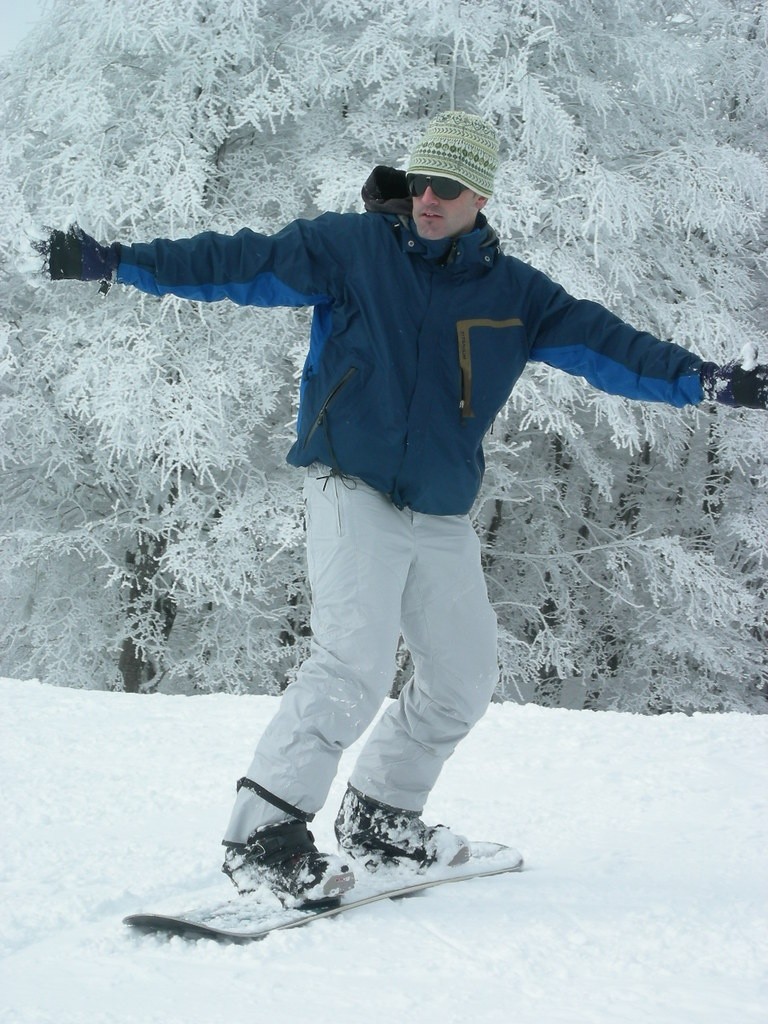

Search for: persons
xmin=24 ymin=111 xmax=768 ymax=909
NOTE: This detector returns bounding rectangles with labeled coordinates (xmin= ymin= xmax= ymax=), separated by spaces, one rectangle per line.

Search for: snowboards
xmin=122 ymin=834 xmax=525 ymax=943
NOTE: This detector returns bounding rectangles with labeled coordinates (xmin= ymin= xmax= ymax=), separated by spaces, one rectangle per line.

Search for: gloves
xmin=701 ymin=342 xmax=768 ymax=409
xmin=28 ymin=215 xmax=116 ymax=296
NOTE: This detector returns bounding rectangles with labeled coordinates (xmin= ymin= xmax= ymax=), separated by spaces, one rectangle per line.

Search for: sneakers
xmin=221 ymin=819 xmax=355 ymax=905
xmin=333 ymin=784 xmax=470 ymax=870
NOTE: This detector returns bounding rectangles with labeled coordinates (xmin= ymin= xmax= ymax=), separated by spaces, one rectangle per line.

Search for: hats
xmin=406 ymin=111 xmax=499 ymax=199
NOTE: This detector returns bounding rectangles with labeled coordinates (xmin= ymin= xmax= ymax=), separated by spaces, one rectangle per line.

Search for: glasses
xmin=407 ymin=174 xmax=469 ymax=199
